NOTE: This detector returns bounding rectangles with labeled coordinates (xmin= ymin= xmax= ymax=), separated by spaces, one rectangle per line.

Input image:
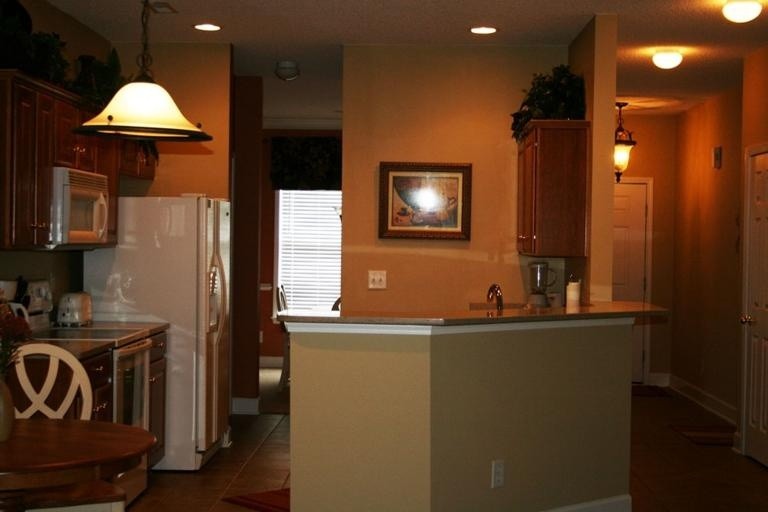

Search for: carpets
xmin=219 ymin=487 xmax=289 ymax=512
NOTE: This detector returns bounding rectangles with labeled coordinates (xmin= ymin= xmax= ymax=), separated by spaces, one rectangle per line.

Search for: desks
xmin=277 ymin=307 xmax=341 ymax=386
xmin=0 ymin=418 xmax=161 ymax=511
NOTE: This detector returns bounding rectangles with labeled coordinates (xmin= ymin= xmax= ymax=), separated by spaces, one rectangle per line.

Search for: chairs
xmin=332 ymin=297 xmax=342 ymax=311
xmin=0 ymin=342 xmax=94 ymax=421
xmin=275 ymin=284 xmax=291 ymax=387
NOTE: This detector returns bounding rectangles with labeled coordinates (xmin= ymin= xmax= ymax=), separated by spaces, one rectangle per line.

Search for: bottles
xmin=566 ymin=280 xmax=581 ymax=307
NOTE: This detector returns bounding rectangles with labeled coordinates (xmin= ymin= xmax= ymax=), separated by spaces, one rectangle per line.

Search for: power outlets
xmin=491 ymin=461 xmax=505 ymax=489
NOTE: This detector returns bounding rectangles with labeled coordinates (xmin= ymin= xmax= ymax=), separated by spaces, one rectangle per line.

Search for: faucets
xmin=487 ymin=310 xmax=504 ymax=320
xmin=487 ymin=283 xmax=503 ymax=310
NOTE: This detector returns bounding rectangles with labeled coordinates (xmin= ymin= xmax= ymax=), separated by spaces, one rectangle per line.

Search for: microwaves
xmin=36 ymin=166 xmax=108 ymax=250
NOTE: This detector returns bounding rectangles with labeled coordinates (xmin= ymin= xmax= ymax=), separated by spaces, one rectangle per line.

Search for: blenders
xmin=527 ymin=261 xmax=557 ymax=308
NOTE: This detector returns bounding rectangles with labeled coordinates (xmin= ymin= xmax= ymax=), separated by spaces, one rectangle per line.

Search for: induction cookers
xmin=32 ymin=328 xmax=149 ymax=347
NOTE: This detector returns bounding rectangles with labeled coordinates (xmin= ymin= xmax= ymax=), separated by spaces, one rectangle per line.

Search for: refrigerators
xmin=83 ymin=196 xmax=230 ymax=471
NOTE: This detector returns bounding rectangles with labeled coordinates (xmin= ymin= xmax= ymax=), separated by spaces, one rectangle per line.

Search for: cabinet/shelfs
xmin=120 ymin=139 xmax=161 ymax=180
xmin=53 ymin=98 xmax=98 ymax=173
xmin=72 ymin=347 xmax=114 ymax=423
xmin=516 ymin=119 xmax=593 ymax=259
xmin=8 ymin=84 xmax=53 ymax=251
xmin=119 ymin=331 xmax=172 ymax=472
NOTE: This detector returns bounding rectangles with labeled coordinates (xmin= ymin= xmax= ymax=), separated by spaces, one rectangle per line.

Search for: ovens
xmin=113 ymin=338 xmax=152 ymax=508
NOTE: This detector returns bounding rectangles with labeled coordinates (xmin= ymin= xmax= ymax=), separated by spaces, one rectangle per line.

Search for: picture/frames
xmin=378 ymin=160 xmax=472 ymax=241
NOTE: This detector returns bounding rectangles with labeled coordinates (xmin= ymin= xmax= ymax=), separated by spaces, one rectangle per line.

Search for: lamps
xmin=650 ymin=46 xmax=686 ymax=70
xmin=71 ymin=0 xmax=214 ymax=143
xmin=722 ymin=0 xmax=765 ymax=25
xmin=614 ymin=100 xmax=636 ymax=184
xmin=274 ymin=58 xmax=302 ymax=83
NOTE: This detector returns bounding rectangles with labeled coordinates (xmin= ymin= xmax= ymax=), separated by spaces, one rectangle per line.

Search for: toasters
xmin=57 ymin=292 xmax=93 ymax=325
